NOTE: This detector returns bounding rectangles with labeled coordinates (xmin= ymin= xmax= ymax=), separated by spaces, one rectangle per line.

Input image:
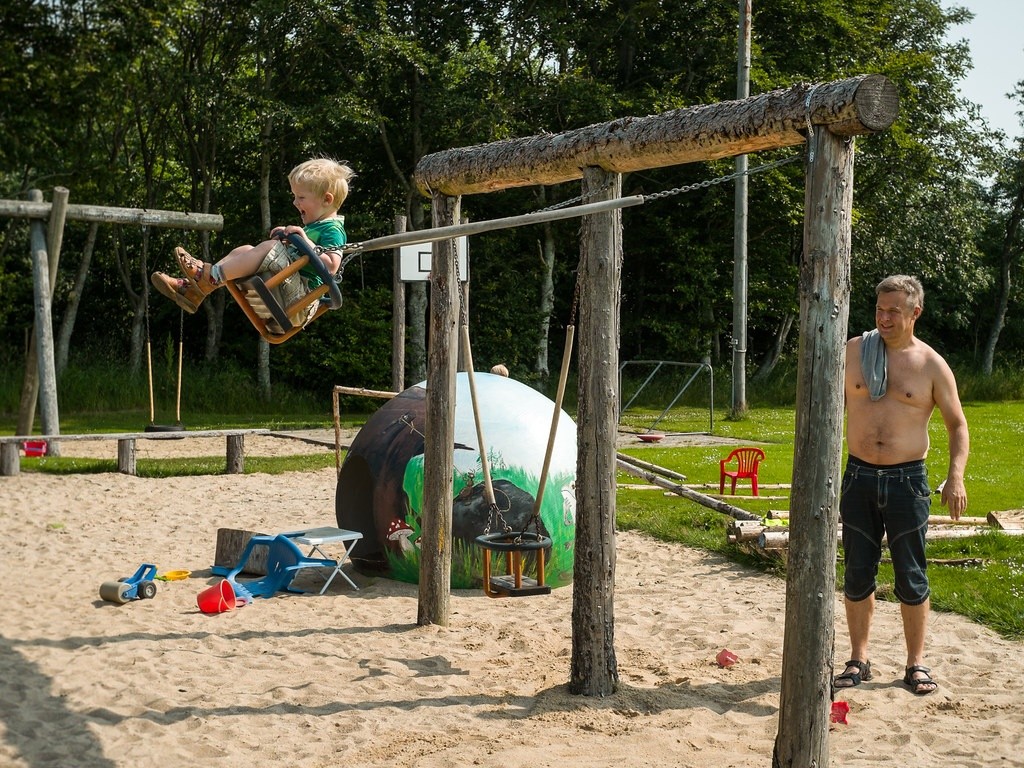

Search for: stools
xmin=289 ymin=526 xmax=363 ymax=595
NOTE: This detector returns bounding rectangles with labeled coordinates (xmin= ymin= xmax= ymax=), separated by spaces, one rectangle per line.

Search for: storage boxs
xmin=197 ymin=579 xmax=236 ymax=613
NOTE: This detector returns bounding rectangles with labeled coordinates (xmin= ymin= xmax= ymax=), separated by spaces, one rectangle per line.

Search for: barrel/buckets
xmin=21 ymin=439 xmax=48 ymax=458
xmin=197 ymin=580 xmax=237 ymax=613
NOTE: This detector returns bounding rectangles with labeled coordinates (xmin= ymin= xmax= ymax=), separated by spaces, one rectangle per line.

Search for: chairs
xmin=720 ymin=448 xmax=765 ymax=496
xmin=226 ymin=530 xmax=338 ymax=605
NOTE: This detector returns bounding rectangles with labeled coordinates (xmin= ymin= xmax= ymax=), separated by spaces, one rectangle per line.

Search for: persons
xmin=835 ymin=276 xmax=972 ymax=695
xmin=148 ymin=152 xmax=358 ymax=336
xmin=491 ymin=364 xmax=510 ymax=379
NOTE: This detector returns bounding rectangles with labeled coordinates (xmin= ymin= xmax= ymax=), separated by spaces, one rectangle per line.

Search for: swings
xmin=450 ymin=202 xmax=583 ymax=597
xmin=142 ymin=226 xmax=191 ymax=440
xmin=225 ymin=152 xmax=807 ymax=343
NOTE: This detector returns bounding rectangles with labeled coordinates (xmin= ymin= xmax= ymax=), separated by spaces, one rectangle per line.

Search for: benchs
xmin=0 ymin=428 xmax=270 ymax=476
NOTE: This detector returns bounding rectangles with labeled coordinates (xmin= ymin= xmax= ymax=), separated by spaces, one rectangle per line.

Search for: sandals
xmin=151 ymin=271 xmax=204 ymax=314
xmin=172 ymin=247 xmax=223 ymax=299
xmin=903 ymin=665 xmax=940 ymax=694
xmin=831 ymin=655 xmax=873 ymax=690
